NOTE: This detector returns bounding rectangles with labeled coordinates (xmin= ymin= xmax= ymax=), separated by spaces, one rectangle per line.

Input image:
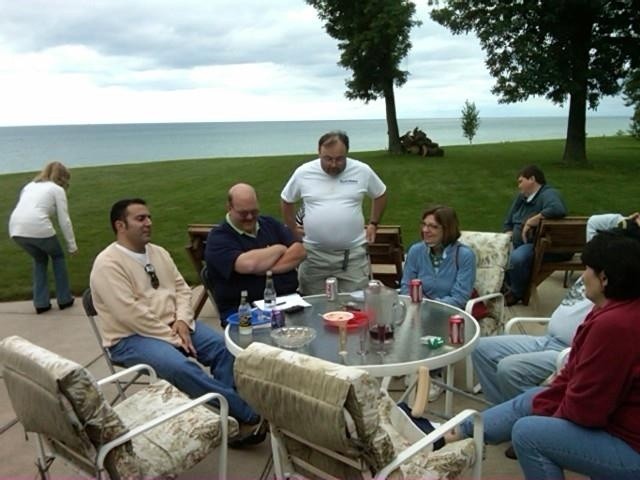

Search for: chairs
xmin=232 ymin=342 xmax=485 ymax=479
xmin=506 ymin=316 xmax=572 ymax=376
xmin=379 ymin=230 xmax=514 ymax=400
xmin=83 ymin=286 xmax=209 ymax=412
xmin=2 ymin=333 xmax=240 ymax=480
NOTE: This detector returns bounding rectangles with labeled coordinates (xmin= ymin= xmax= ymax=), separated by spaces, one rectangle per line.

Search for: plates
xmin=346 ymin=311 xmax=374 ymax=328
xmin=351 ymin=291 xmax=365 ymax=302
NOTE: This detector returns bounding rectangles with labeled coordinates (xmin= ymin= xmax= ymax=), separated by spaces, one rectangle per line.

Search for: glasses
xmin=145 ymin=264 xmax=159 ymax=289
xmin=419 ymin=222 xmax=440 ymax=230
xmin=232 ymin=207 xmax=259 ymax=218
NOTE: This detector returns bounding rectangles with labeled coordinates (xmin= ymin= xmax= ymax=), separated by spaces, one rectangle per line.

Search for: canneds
xmin=271 ymin=309 xmax=286 ymax=331
xmin=448 ymin=315 xmax=464 ymax=345
xmin=368 ymin=279 xmax=382 ymax=295
xmin=408 ymin=279 xmax=423 ymax=303
xmin=325 ymin=277 xmax=338 ymax=302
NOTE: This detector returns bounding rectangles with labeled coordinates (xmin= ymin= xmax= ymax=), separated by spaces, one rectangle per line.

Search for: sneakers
xmin=427 ymin=373 xmax=444 ymax=402
xmin=398 ymin=402 xmax=445 ymax=451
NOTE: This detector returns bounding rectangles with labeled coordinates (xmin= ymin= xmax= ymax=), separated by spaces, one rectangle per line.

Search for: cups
xmin=375 ymin=322 xmax=388 ymax=355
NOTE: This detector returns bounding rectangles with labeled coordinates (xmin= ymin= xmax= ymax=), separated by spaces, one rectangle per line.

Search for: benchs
xmin=519 ymin=213 xmax=587 ymax=308
xmin=185 ymin=223 xmax=405 ymax=289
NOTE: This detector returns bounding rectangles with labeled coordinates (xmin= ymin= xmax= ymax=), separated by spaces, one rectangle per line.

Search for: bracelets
xmin=369 ymin=221 xmax=378 ymax=228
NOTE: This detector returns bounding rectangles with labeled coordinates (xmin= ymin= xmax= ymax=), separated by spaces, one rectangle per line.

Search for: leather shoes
xmin=229 ymin=423 xmax=266 ymax=447
xmin=59 ymin=298 xmax=74 ymax=309
xmin=504 ymin=291 xmax=520 ymax=306
xmin=37 ymin=304 xmax=51 ymax=313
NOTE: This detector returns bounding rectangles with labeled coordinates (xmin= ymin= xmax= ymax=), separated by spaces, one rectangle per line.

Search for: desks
xmin=225 ymin=293 xmax=479 ymax=479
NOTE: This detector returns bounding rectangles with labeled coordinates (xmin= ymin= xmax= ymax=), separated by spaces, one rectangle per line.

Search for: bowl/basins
xmin=270 ymin=326 xmax=317 ymax=347
xmin=321 ymin=310 xmax=354 ymax=327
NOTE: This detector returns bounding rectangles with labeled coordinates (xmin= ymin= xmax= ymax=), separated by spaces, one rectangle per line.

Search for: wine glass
xmin=357 ymin=318 xmax=370 ymax=355
xmin=337 ymin=318 xmax=349 ymax=357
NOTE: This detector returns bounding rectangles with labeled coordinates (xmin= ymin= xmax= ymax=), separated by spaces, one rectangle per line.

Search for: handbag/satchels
xmin=453 ymin=244 xmax=488 ymax=320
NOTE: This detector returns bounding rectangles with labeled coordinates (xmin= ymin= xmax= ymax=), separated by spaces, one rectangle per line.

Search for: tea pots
xmin=365 ymin=279 xmax=406 ymax=343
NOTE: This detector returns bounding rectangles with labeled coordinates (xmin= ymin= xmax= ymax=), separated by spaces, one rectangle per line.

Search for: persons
xmin=502 ymin=162 xmax=567 ymax=307
xmin=399 ymin=204 xmax=478 ymax=403
xmin=278 ymin=130 xmax=390 ymax=295
xmin=88 ymin=198 xmax=269 ymax=450
xmin=469 ymin=212 xmax=640 ymax=459
xmin=205 ymin=180 xmax=309 ymax=327
xmin=8 ymin=161 xmax=79 ymax=316
xmin=445 ymin=224 xmax=640 ymax=480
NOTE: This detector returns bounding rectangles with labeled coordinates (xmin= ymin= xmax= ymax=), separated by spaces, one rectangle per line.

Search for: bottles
xmin=263 ymin=270 xmax=277 ymax=302
xmin=237 ymin=290 xmax=253 ymax=334
